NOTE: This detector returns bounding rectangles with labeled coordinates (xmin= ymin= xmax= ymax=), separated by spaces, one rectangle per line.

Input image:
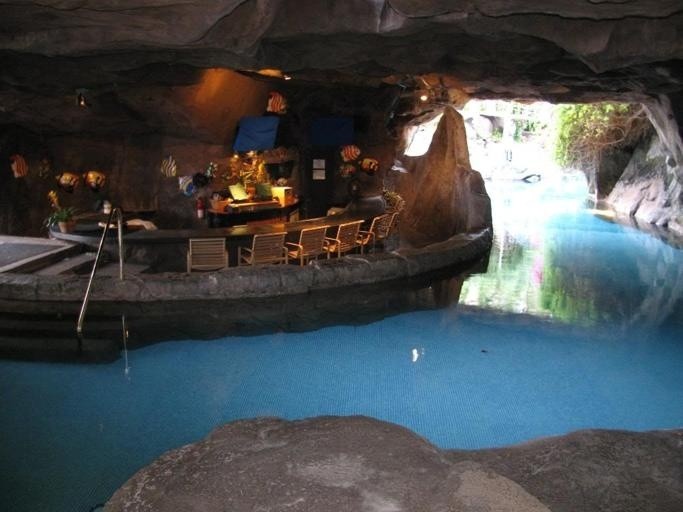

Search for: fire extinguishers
xmin=196 ymin=196 xmax=205 ymax=218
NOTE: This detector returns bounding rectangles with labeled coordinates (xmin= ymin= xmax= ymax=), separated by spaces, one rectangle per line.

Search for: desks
xmin=206 ymin=196 xmax=299 ymax=222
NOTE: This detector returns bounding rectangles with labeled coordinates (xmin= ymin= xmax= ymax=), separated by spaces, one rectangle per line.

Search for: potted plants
xmin=43 ymin=206 xmax=82 ymax=234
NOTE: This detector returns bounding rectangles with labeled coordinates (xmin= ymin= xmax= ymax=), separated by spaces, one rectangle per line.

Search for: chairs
xmin=326 ymin=219 xmax=365 ymax=258
xmin=237 ymin=231 xmax=289 ymax=269
xmin=360 ymin=215 xmax=397 ymax=255
xmin=285 ymin=224 xmax=330 ymax=266
xmin=186 ymin=238 xmax=229 ymax=273
